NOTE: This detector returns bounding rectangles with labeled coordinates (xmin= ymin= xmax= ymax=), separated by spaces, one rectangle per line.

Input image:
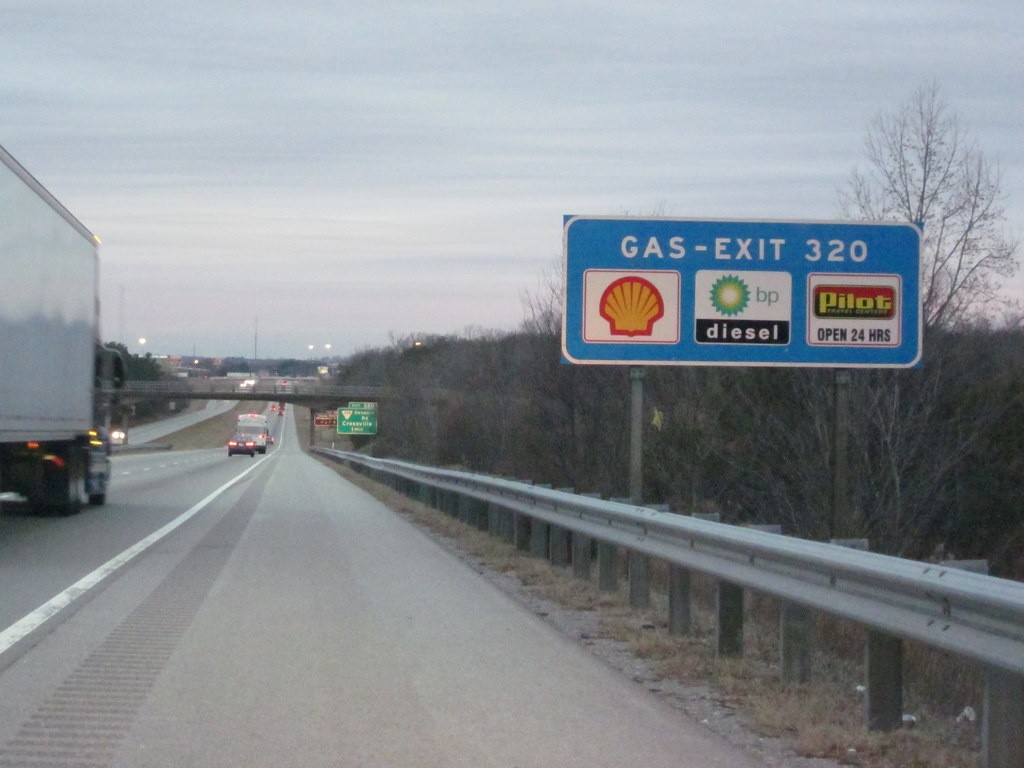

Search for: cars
xmin=228 ymin=435 xmax=255 ymax=457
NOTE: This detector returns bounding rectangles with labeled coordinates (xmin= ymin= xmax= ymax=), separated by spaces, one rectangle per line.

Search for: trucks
xmin=1 ymin=145 xmax=127 ymax=517
xmin=236 ymin=414 xmax=268 ymax=454
